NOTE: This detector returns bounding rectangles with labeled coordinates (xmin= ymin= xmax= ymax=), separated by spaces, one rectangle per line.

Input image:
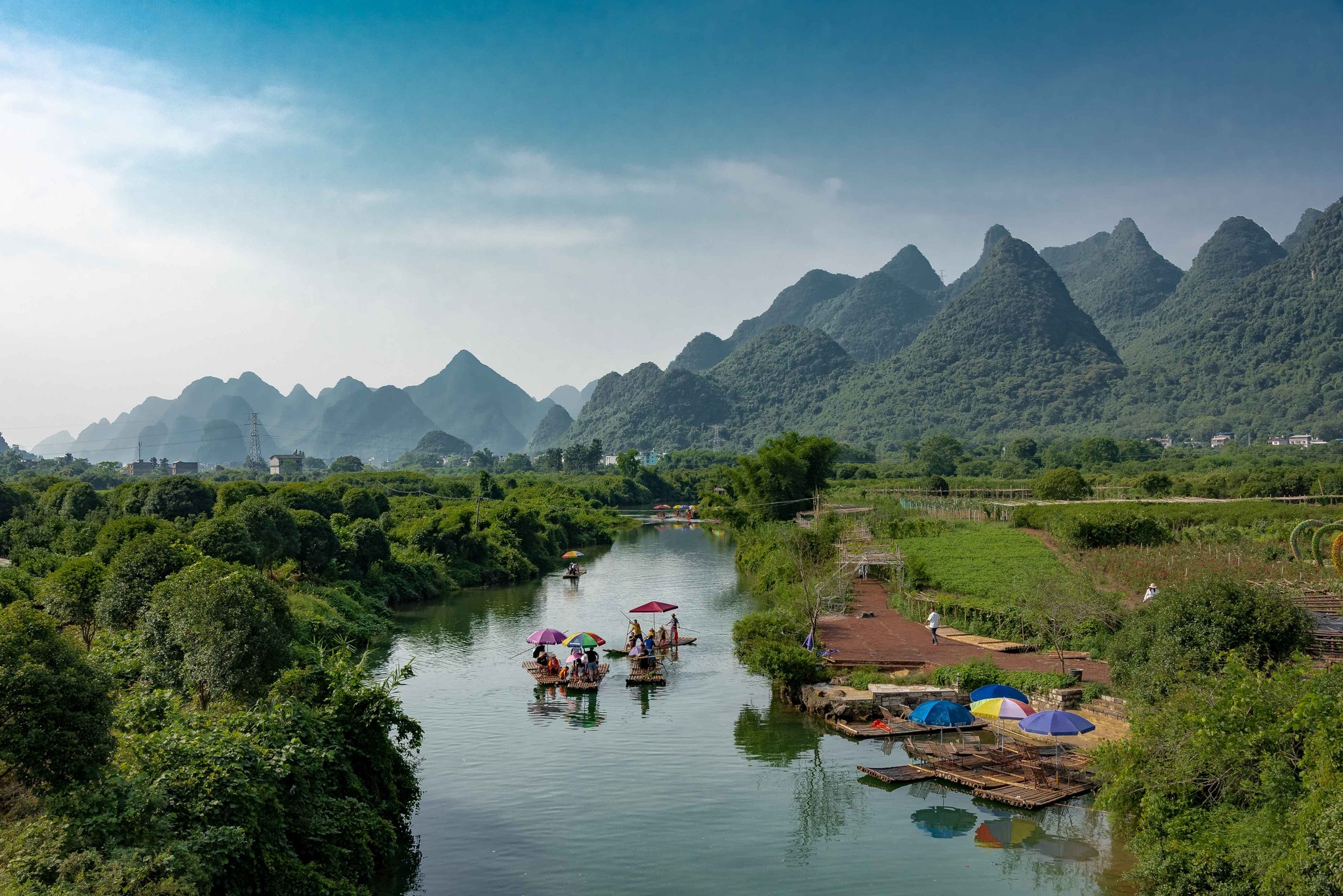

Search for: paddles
xmin=661 ymin=660 xmax=668 ymax=674
xmin=620 ymin=610 xmax=673 ymax=663
xmin=508 ymin=630 xmax=568 ymax=660
xmin=618 ymin=654 xmax=671 ymax=662
xmin=982 ymin=776 xmax=1037 ymax=791
xmin=577 ymin=567 xmax=580 ymax=576
xmin=1052 ymin=758 xmax=1082 ymax=768
xmin=1054 ymin=803 xmax=1137 ymax=817
xmin=642 ymin=619 xmax=700 ymax=633
xmin=623 ymin=621 xmax=630 ymax=650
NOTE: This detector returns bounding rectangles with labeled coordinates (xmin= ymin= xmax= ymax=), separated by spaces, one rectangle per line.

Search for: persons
xmin=861 ymin=564 xmax=869 ymax=581
xmin=643 ymin=634 xmax=658 ymax=652
xmin=857 ymin=567 xmax=864 ymax=585
xmin=634 ymin=644 xmax=651 ymax=680
xmin=569 ymin=562 xmax=578 ymax=575
xmin=628 ymin=632 xmax=637 ymax=648
xmin=656 ymin=509 xmax=697 ymax=518
xmin=657 ymin=624 xmax=667 ymax=645
xmin=666 ymin=612 xmax=680 ymax=643
xmin=1142 ymin=583 xmax=1160 ymax=603
xmin=647 ymin=650 xmax=664 ymax=673
xmin=533 ymin=644 xmax=600 ymax=684
xmin=923 ymin=607 xmax=942 ymax=646
xmin=626 ymin=619 xmax=643 ymax=644
xmin=644 ymin=628 xmax=656 ymax=643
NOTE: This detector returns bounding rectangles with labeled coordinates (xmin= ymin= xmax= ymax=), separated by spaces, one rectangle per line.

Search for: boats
xmin=831 ymin=704 xmax=1117 ymax=809
xmin=650 ymin=513 xmax=703 ymax=519
xmin=561 ymin=568 xmax=586 ymax=579
xmin=518 ymin=635 xmax=698 ymax=690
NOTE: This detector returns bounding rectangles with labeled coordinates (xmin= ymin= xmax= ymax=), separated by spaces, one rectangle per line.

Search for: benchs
xmin=1014 ymin=741 xmax=1052 ymax=767
xmin=927 ymin=740 xmax=963 ymax=768
xmin=988 ymin=724 xmax=1017 ymax=749
xmin=984 ymin=746 xmax=1024 ymax=772
xmin=1038 ymin=759 xmax=1081 ymax=788
xmin=878 ymin=705 xmax=908 ymax=731
xmin=1017 ymin=763 xmax=1060 ymax=794
xmin=906 ymin=744 xmax=943 ymax=770
xmin=956 ymin=726 xmax=981 ymax=752
xmin=899 ymin=704 xmax=925 ymax=728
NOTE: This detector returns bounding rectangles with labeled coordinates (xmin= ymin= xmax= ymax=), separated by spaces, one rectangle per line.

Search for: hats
xmin=650 ymin=628 xmax=653 ymax=631
xmin=551 ymin=653 xmax=557 ymax=658
xmin=659 ymin=625 xmax=664 ymax=628
xmin=670 ymin=613 xmax=676 ymax=616
xmin=539 ymin=651 xmax=545 ymax=658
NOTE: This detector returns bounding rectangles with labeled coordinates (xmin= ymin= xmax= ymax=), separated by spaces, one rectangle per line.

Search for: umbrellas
xmin=562 ymin=631 xmax=607 ymax=666
xmin=628 ymin=600 xmax=679 ymax=638
xmin=969 ymin=683 xmax=1030 ymax=741
xmin=565 ymin=653 xmax=585 ymax=664
xmin=653 ymin=504 xmax=699 ymax=511
xmin=969 ymin=697 xmax=1037 ymax=762
xmin=1017 ymin=709 xmax=1096 ymax=788
xmin=907 ymin=698 xmax=978 ymax=755
xmin=562 ymin=550 xmax=585 ymax=566
xmin=628 ymin=644 xmax=649 ymax=657
xmin=532 ymin=645 xmax=546 ymax=659
xmin=526 ymin=627 xmax=569 ymax=660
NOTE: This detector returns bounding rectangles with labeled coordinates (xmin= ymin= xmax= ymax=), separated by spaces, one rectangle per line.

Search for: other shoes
xmin=931 ymin=641 xmax=938 ymax=645
